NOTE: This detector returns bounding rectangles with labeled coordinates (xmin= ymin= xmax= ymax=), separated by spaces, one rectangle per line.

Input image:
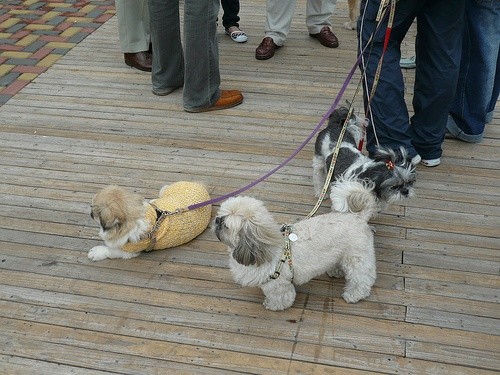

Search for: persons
xmin=355 ymin=0 xmax=464 ymax=168
xmin=444 ymin=0 xmax=500 ymax=144
xmin=114 ymin=0 xmax=153 ymax=73
xmin=148 ymin=0 xmax=244 ymax=113
xmin=255 ymin=0 xmax=340 ymax=61
xmin=215 ymin=0 xmax=248 ymax=43
xmin=347 ymin=0 xmax=364 ymax=31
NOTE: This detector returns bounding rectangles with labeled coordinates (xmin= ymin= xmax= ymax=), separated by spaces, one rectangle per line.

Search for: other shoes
xmin=394 ymin=147 xmax=422 ymax=166
xmin=224 ymin=27 xmax=248 ymax=43
xmin=419 ymin=157 xmax=441 ymax=167
xmin=443 ymin=128 xmax=459 ymax=140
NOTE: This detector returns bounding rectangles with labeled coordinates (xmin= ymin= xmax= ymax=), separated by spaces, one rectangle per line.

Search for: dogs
xmin=212 ymin=174 xmax=379 ymax=310
xmin=312 ymin=107 xmax=416 ymax=223
xmin=343 ymin=0 xmax=360 ymax=30
xmin=88 ymin=180 xmax=212 ymax=261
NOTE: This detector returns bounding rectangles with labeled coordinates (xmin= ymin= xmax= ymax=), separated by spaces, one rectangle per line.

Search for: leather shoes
xmin=124 ymin=51 xmax=153 ymax=71
xmin=182 ymin=89 xmax=244 ymax=113
xmin=255 ymin=37 xmax=281 ymax=60
xmin=309 ymin=27 xmax=339 ymax=47
xmin=151 ymin=83 xmax=180 ymax=96
